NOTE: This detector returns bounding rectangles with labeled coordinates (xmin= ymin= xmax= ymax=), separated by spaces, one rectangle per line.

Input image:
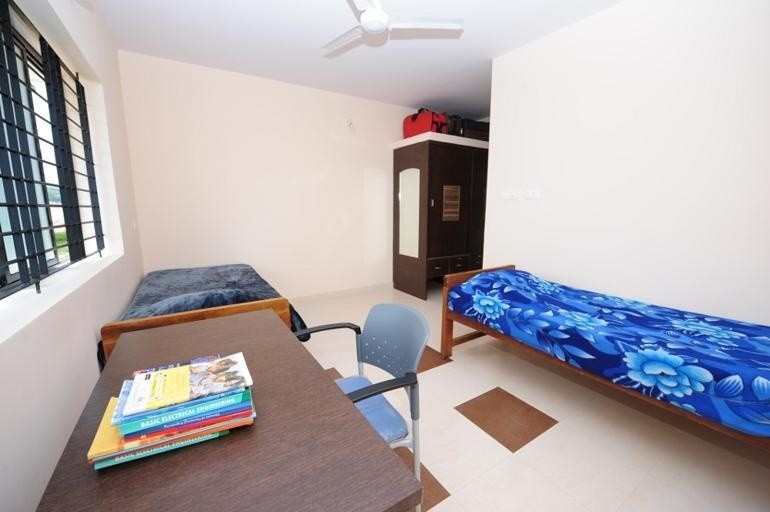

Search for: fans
xmin=316 ymin=0 xmax=465 ymax=61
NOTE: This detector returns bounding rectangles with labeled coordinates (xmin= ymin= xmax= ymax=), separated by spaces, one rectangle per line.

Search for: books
xmin=85 ymin=349 xmax=258 ymax=472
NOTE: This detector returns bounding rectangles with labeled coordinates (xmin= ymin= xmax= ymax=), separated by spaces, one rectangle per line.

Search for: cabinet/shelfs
xmin=387 ymin=133 xmax=490 ymax=300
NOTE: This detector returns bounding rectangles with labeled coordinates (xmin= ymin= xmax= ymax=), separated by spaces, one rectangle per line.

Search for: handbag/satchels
xmin=403 ymin=108 xmax=447 ymax=139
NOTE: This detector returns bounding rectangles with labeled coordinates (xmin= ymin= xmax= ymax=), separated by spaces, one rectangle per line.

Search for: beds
xmin=99 ymin=241 xmax=314 ymax=372
xmin=436 ymin=263 xmax=770 ymax=450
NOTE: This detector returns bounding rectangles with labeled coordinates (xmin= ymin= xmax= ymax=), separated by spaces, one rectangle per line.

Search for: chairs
xmin=293 ymin=301 xmax=431 ymax=511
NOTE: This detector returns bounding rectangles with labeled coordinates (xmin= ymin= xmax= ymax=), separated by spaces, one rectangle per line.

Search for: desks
xmin=1 ymin=307 xmax=441 ymax=511
